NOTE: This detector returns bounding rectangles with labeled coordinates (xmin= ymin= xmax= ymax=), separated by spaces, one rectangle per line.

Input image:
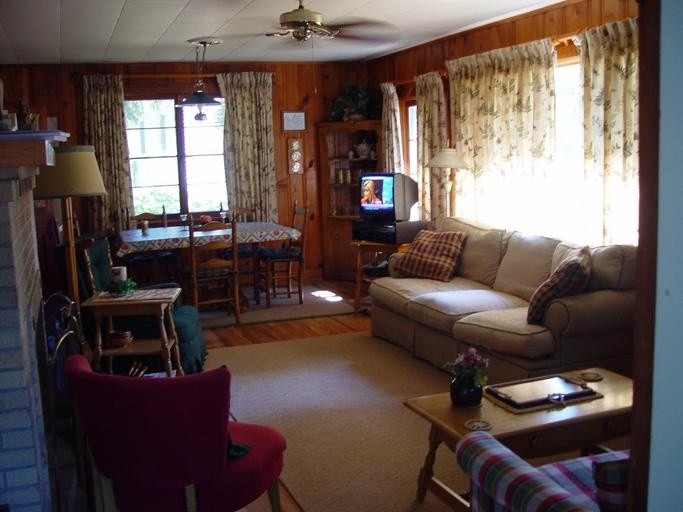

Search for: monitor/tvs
xmin=358 ymin=172 xmax=419 ymax=221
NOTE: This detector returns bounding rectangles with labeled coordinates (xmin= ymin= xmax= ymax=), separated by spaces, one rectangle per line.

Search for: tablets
xmin=485 ymin=373 xmax=593 ymax=408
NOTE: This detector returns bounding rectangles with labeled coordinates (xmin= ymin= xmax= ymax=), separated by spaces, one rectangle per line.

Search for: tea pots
xmin=353 ymin=140 xmax=372 ymax=158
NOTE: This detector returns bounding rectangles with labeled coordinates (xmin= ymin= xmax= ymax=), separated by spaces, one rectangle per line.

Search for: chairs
xmin=52 ymin=199 xmax=310 ymax=325
xmin=454 ymin=430 xmax=631 ymax=511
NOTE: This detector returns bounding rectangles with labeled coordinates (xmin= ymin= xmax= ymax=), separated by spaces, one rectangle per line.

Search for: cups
xmin=142 ymin=220 xmax=150 ymax=235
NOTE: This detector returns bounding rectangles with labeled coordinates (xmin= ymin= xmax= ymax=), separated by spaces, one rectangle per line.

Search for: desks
xmin=349 ymin=238 xmax=407 ymax=317
xmin=404 ymin=367 xmax=632 ymax=512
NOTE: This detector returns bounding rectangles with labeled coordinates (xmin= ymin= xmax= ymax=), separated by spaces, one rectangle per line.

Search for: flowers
xmin=439 ymin=342 xmax=495 ymax=388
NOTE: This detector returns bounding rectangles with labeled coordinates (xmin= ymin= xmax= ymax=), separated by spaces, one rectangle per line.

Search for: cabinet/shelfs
xmin=315 ymin=121 xmax=387 ymax=280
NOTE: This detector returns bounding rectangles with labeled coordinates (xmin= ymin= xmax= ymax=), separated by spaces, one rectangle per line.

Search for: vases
xmin=449 ymin=373 xmax=483 ymax=407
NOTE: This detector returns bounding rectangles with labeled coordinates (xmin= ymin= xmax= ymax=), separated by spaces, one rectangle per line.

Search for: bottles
xmin=337 ymin=168 xmax=352 ymax=184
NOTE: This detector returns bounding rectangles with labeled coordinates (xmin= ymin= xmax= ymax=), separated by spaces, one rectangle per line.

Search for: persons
xmin=360 ymin=179 xmax=382 ymax=204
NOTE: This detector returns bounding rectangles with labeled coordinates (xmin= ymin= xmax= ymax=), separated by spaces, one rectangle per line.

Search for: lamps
xmin=173 ymin=35 xmax=221 ymax=122
xmin=428 ymin=148 xmax=466 ymax=217
xmin=32 ymin=143 xmax=104 ymax=364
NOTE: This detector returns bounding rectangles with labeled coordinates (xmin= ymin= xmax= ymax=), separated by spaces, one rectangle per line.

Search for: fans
xmin=190 ymin=1 xmax=394 ymax=50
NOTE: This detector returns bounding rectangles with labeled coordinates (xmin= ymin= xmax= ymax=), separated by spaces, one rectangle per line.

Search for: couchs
xmin=367 ymin=215 xmax=638 ymax=392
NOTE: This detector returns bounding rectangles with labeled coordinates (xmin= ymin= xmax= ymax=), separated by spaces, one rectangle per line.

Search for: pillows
xmin=526 ymin=244 xmax=592 ymax=324
xmin=394 ymin=229 xmax=467 ymax=281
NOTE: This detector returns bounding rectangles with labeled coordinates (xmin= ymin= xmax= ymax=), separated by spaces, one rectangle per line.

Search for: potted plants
xmin=326 ymin=86 xmax=380 ymax=120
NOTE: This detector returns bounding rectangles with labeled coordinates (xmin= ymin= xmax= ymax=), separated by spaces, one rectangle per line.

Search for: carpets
xmin=200 ymin=331 xmax=581 ymax=512
xmin=187 ymin=283 xmax=356 ymax=328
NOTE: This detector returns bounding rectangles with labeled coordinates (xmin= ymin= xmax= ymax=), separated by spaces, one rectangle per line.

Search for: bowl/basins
xmin=108 ymin=330 xmax=133 ymax=349
xmin=348 ymin=113 xmax=365 ymax=121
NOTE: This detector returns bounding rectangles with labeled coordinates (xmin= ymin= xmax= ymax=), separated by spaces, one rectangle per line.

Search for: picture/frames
xmin=280 ymin=109 xmax=304 ymax=134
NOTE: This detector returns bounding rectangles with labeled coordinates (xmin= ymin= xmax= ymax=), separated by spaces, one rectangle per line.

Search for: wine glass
xmin=180 ymin=214 xmax=188 ymax=232
xmin=220 ymin=212 xmax=227 ymax=224
